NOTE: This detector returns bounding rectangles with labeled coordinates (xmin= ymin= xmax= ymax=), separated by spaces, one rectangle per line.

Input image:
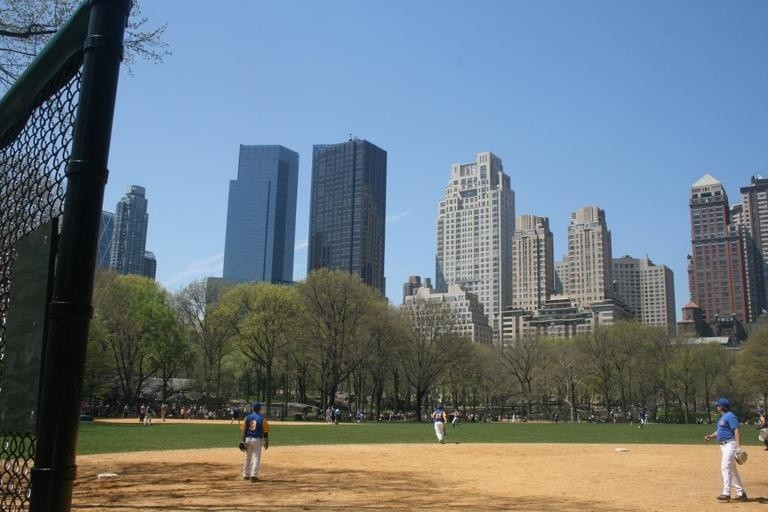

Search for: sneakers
xmin=242 ymin=477 xmax=262 ymax=482
xmin=716 ymin=492 xmax=748 ymax=502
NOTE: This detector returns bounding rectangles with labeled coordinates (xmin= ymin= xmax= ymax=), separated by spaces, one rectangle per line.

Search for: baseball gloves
xmin=735 ymin=448 xmax=748 ymax=465
xmin=755 ymin=423 xmax=762 ymax=430
xmin=239 ymin=441 xmax=246 ymax=452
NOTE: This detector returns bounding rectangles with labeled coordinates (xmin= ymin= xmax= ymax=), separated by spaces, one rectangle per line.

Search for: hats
xmin=713 ymin=397 xmax=731 ymax=409
xmin=252 ymin=402 xmax=260 ymax=407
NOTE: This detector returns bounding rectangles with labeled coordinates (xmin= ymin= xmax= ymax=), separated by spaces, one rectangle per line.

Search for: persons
xmin=588 ymin=407 xmax=681 ymax=429
xmin=755 ymin=408 xmax=768 ymax=452
xmin=705 ymin=398 xmax=748 ymax=503
xmin=239 ymin=401 xmax=269 ymax=482
xmin=80 ymin=399 xmax=248 ymax=427
xmin=697 ymin=415 xmax=703 ymax=425
xmin=302 ymin=403 xmax=452 ymax=444
xmin=272 ymin=407 xmax=281 ymax=421
xmin=464 ymin=410 xmax=559 ymax=424
xmin=449 ymin=408 xmax=461 ymax=429
